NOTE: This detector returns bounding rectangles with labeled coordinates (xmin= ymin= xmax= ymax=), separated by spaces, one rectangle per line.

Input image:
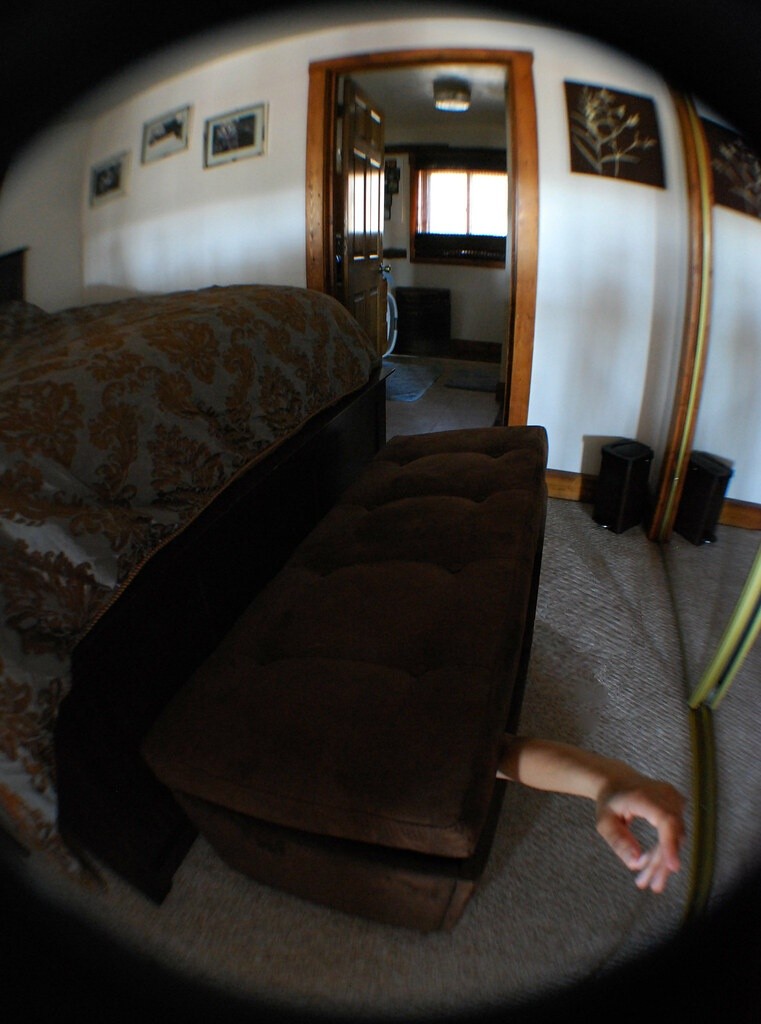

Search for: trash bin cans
xmin=673 ymin=449 xmax=734 ymax=547
xmin=591 ymin=438 xmax=656 ymax=536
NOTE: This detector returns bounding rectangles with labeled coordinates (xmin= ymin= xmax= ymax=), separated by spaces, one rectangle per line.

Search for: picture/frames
xmin=88 ymin=148 xmax=130 ymax=205
xmin=202 ymin=100 xmax=265 ymax=169
xmin=138 ymin=104 xmax=189 ymax=164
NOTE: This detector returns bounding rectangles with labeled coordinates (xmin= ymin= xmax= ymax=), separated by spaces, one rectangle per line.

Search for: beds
xmin=0 ymin=247 xmax=396 ymax=890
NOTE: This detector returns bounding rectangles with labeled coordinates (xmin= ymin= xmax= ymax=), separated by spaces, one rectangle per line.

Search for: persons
xmin=495 ymin=738 xmax=687 ymax=895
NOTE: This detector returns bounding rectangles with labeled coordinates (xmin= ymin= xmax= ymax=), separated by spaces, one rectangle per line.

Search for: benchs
xmin=146 ymin=426 xmax=546 ymax=938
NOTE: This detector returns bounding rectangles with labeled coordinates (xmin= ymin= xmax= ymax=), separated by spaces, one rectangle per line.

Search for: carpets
xmin=443 ymin=367 xmax=500 ymax=390
xmin=381 ymin=361 xmax=441 ymax=403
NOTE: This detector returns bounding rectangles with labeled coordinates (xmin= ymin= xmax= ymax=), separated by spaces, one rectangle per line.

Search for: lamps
xmin=432 ymin=82 xmax=471 ymax=112
